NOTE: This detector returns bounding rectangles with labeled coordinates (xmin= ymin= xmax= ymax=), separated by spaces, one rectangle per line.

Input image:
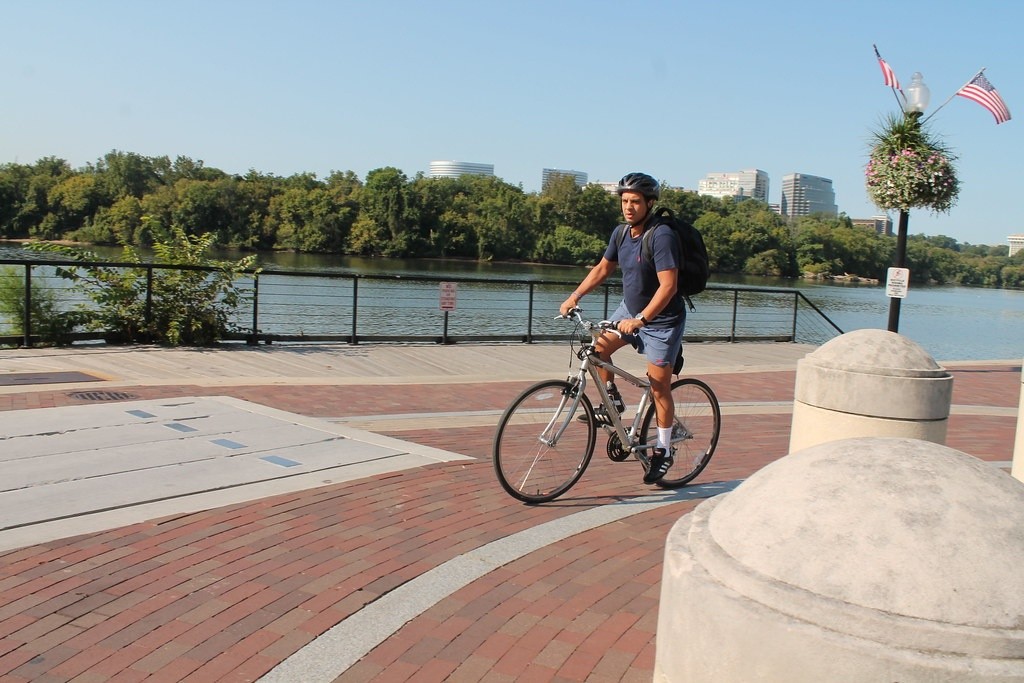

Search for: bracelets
xmin=572 ymin=291 xmax=582 ymax=298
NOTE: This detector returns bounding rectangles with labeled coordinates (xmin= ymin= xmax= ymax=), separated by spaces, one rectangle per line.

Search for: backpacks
xmin=654 ymin=206 xmax=710 ymax=294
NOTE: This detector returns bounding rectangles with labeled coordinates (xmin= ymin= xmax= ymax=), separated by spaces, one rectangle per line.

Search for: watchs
xmin=635 ymin=312 xmax=648 ymax=326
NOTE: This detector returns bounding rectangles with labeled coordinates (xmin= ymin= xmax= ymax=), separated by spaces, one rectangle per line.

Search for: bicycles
xmin=493 ymin=305 xmax=720 ymax=507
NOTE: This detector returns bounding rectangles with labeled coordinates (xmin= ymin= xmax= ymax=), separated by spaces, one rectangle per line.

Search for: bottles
xmin=605 ymin=380 xmax=626 ymax=414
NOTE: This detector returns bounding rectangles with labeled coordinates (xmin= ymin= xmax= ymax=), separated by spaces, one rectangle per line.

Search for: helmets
xmin=617 ymin=172 xmax=658 ymax=203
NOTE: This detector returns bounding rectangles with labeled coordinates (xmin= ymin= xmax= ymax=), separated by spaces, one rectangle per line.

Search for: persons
xmin=559 ymin=172 xmax=687 ymax=483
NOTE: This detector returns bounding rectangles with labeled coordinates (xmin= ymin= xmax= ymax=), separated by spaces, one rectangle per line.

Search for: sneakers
xmin=575 ymin=403 xmax=621 ymax=426
xmin=642 ymin=445 xmax=673 ymax=484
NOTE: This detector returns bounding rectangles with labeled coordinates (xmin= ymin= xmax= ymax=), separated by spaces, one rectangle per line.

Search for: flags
xmin=873 ymin=45 xmax=907 ymax=104
xmin=957 ymin=70 xmax=1013 ymax=126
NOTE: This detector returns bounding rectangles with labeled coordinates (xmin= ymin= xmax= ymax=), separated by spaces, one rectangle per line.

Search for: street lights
xmin=887 ymin=71 xmax=933 ymax=332
